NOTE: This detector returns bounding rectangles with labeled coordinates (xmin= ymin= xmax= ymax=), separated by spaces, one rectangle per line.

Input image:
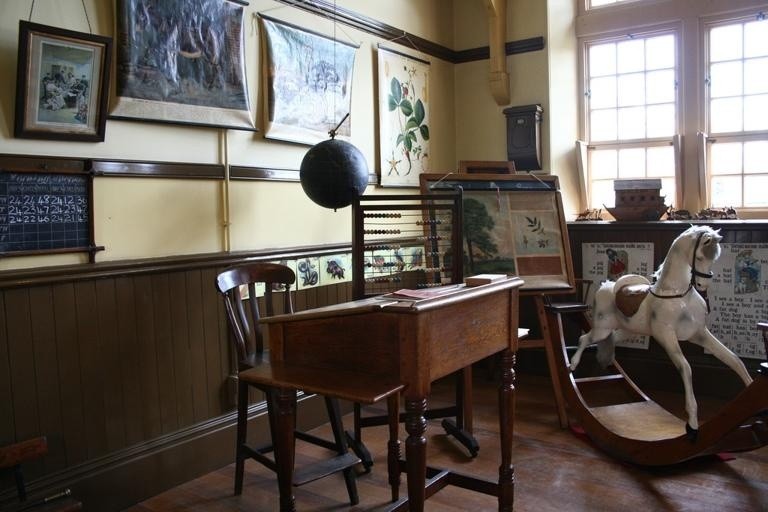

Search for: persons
xmin=39 ymin=70 xmax=88 ymax=122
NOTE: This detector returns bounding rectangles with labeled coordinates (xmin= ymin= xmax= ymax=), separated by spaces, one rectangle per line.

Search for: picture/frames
xmin=14 ymin=19 xmax=114 ymax=143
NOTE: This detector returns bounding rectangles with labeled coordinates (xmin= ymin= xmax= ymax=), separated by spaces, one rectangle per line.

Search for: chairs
xmin=214 ymin=263 xmax=359 ymax=511
xmin=0 ymin=436 xmax=83 ymax=512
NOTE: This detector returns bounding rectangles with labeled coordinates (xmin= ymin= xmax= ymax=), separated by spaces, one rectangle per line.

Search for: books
xmin=374 ymin=288 xmax=443 ymax=302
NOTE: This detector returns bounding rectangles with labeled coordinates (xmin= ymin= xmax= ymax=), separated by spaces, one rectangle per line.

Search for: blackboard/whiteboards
xmin=0 ymin=170 xmax=96 ymax=257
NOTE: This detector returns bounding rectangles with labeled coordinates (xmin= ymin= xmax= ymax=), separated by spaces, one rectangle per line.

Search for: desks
xmin=258 ymin=274 xmax=524 ymax=512
